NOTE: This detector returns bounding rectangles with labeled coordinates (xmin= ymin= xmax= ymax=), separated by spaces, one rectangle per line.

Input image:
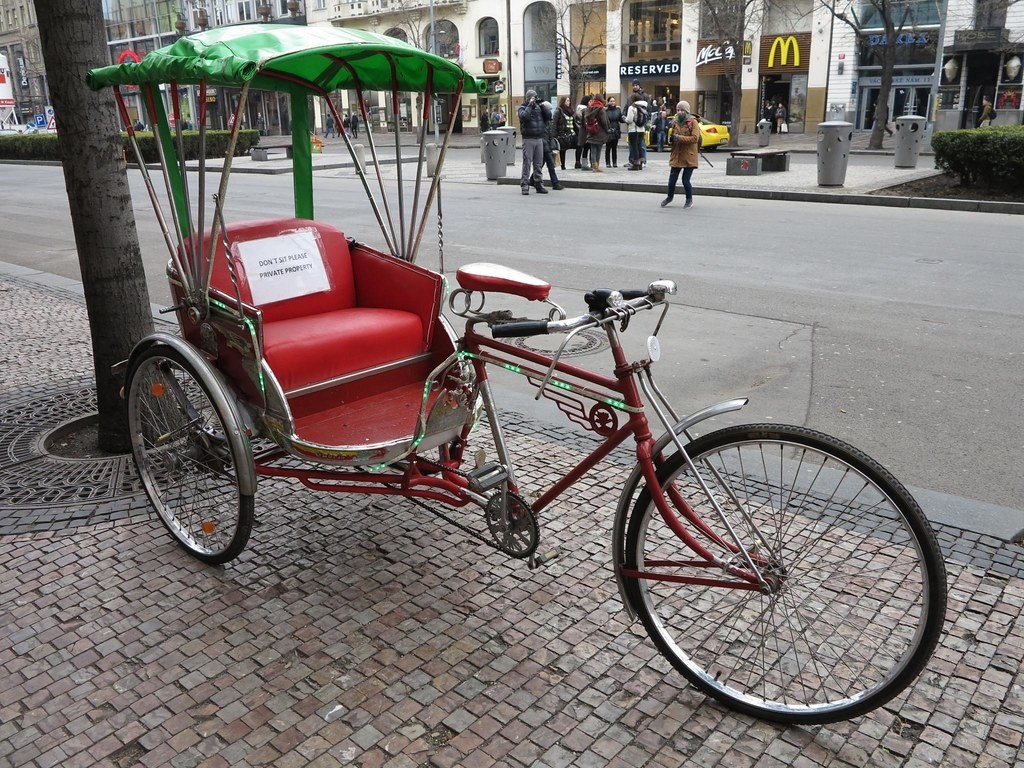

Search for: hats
xmin=632 ymin=79 xmax=640 ymax=86
xmin=526 ymin=90 xmax=538 ymax=98
xmin=676 ymin=101 xmax=691 ymax=114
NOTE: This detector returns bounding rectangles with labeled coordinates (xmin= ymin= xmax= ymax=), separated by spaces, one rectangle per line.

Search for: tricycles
xmin=83 ymin=22 xmax=950 ymax=725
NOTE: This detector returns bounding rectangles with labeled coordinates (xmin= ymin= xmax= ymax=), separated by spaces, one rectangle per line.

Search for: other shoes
xmin=642 ymin=163 xmax=646 ymax=167
xmin=552 ymin=184 xmax=564 ymax=190
xmin=613 ymin=161 xmax=617 ymax=167
xmin=561 ymin=165 xmax=566 ymax=170
xmin=684 ymin=200 xmax=693 ymax=209
xmin=536 ymin=188 xmax=548 ymax=193
xmin=522 ymin=187 xmax=529 ymax=195
xmin=624 ymin=163 xmax=633 ymax=167
xmin=606 ymin=161 xmax=611 ymax=167
xmin=575 ymin=164 xmax=581 ymax=168
xmin=890 ymin=131 xmax=893 ymax=136
xmin=628 ymin=165 xmax=639 ymax=170
xmin=661 ymin=197 xmax=672 ymax=206
xmin=639 ymin=165 xmax=642 ymax=170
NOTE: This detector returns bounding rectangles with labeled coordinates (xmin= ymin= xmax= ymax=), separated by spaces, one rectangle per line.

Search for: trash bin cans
xmin=817 ymin=121 xmax=852 ymax=188
xmin=894 ymin=115 xmax=926 ymax=170
xmin=758 ymin=122 xmax=771 ymax=146
xmin=482 ymin=130 xmax=508 ymax=181
xmin=496 ymin=126 xmax=517 ymax=166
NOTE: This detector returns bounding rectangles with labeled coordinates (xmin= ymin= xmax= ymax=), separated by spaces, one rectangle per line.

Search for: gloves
xmin=670 ymin=134 xmax=678 ymax=142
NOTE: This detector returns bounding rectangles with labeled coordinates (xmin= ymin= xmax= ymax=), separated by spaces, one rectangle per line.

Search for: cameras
xmin=533 ymin=96 xmax=544 ymax=103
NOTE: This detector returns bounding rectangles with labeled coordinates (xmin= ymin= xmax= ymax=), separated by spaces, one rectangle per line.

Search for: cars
xmin=644 ymin=113 xmax=732 ymax=151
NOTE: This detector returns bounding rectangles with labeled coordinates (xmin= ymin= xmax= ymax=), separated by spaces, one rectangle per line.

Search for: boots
xmin=581 ymin=158 xmax=593 ymax=170
xmin=592 ymin=161 xmax=603 ymax=172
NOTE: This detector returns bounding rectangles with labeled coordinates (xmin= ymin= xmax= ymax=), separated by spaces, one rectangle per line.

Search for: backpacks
xmin=632 ymin=104 xmax=648 ymax=127
xmin=496 ymin=114 xmax=499 ymax=120
xmin=585 ymin=107 xmax=601 ymax=133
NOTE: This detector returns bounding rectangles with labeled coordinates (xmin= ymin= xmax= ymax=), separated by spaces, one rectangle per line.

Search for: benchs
xmin=251 ymin=145 xmax=292 ymax=160
xmin=178 ymin=218 xmax=427 ymax=391
xmin=726 ymin=148 xmax=793 ymax=175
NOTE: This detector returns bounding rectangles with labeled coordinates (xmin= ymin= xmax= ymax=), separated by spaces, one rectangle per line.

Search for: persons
xmin=133 ymin=113 xmax=265 ymax=136
xmin=323 ymin=114 xmax=335 ymax=138
xmin=764 ymin=102 xmax=786 ymax=134
xmin=517 ymin=89 xmax=548 ymax=195
xmin=480 ymin=108 xmax=505 ymax=132
xmin=662 ymin=97 xmax=667 ymax=105
xmin=530 ymin=101 xmax=564 ymax=190
xmin=551 ymin=78 xmax=669 ymax=172
xmin=978 ymin=96 xmax=992 ymax=127
xmin=335 ymin=114 xmax=351 ymax=139
xmin=871 ymin=101 xmax=893 ymax=136
xmin=652 ymin=100 xmax=658 ymax=112
xmin=661 ymin=100 xmax=699 ymax=209
xmin=351 ymin=113 xmax=359 ymax=138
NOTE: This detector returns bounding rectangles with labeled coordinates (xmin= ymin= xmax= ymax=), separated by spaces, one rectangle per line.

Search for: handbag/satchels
xmin=781 ymin=121 xmax=788 ymax=132
xmin=552 ymin=138 xmax=562 ymax=166
